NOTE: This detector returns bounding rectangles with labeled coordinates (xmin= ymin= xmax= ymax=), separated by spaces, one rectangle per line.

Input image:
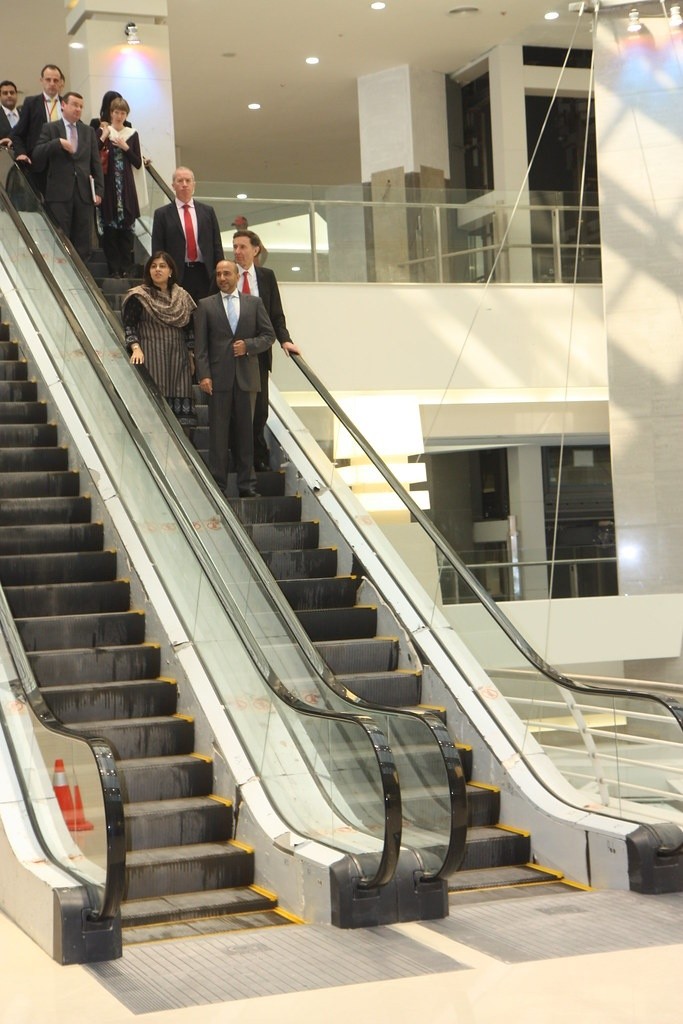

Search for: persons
xmin=122 ymin=251 xmax=195 ymax=445
xmin=152 ymin=166 xmax=225 ymax=302
xmin=1 ymin=72 xmax=64 ymax=148
xmin=96 ymin=98 xmax=142 ymax=278
xmin=195 ymin=261 xmax=276 ymax=498
xmin=32 ymin=92 xmax=105 ymax=264
xmin=91 ymin=91 xmax=152 ymax=166
xmin=205 ymin=230 xmax=300 ymax=472
xmin=0 ymin=81 xmax=22 ymax=145
xmin=232 ymin=216 xmax=269 ymax=266
xmin=12 ymin=64 xmax=64 ymax=194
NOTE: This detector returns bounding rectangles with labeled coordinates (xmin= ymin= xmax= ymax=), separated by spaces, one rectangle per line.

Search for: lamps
xmin=123 ymin=21 xmax=141 ymax=45
xmin=668 ymin=3 xmax=683 ymax=27
xmin=626 ymin=9 xmax=642 ymax=32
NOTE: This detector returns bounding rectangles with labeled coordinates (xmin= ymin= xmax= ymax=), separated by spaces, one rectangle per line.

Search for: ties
xmin=50 ymin=97 xmax=58 ymax=121
xmin=9 ymin=111 xmax=16 ymax=128
xmin=242 ymin=271 xmax=251 ymax=295
xmin=226 ymin=295 xmax=238 ymax=335
xmin=68 ymin=123 xmax=78 ymax=153
xmin=183 ymin=204 xmax=197 ymax=261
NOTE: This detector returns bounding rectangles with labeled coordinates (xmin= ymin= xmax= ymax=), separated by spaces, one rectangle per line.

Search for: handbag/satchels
xmin=100 ymin=125 xmax=109 ymax=176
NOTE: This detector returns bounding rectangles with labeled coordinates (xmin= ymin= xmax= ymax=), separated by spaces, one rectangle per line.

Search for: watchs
xmin=132 ymin=345 xmax=140 ymax=350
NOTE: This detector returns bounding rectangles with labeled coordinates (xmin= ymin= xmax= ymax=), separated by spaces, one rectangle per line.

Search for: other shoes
xmin=254 ymin=458 xmax=272 ymax=472
xmin=238 ymin=488 xmax=261 ymax=500
xmin=111 ymin=270 xmax=131 ymax=279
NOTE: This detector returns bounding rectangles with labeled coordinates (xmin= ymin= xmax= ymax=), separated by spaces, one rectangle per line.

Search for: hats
xmin=231 ymin=217 xmax=247 ymax=227
